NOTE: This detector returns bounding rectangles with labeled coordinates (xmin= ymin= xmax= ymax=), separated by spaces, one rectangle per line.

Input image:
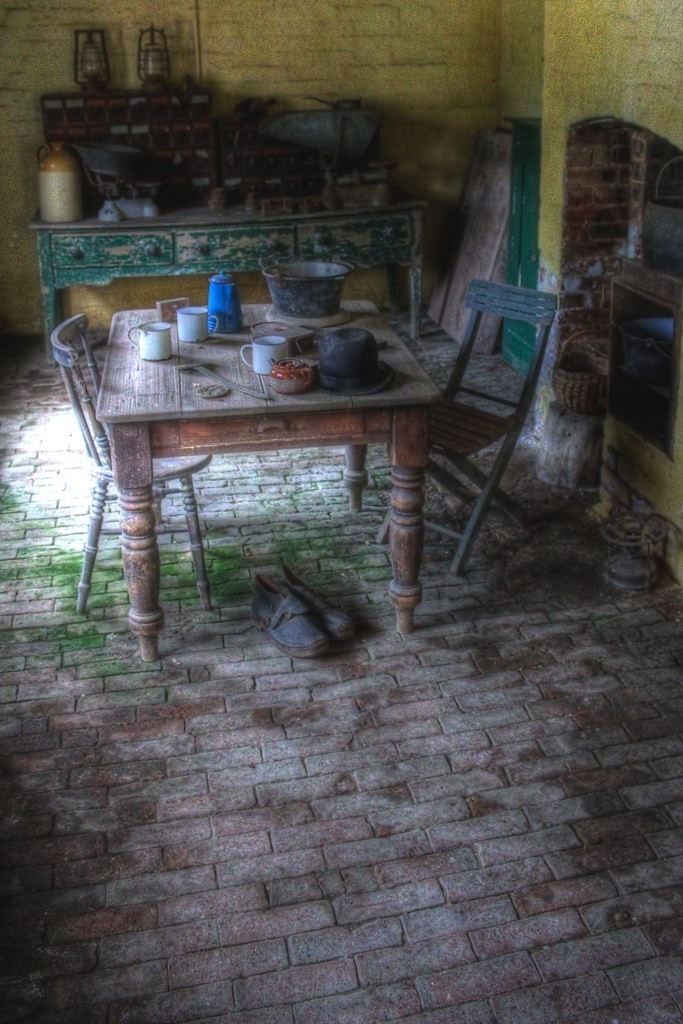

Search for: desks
xmin=95 ymin=300 xmax=443 ymax=664
xmin=25 ymin=186 xmax=429 ymax=339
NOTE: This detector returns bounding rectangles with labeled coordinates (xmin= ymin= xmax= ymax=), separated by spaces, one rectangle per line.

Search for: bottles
xmin=37 ymin=141 xmax=84 ymax=224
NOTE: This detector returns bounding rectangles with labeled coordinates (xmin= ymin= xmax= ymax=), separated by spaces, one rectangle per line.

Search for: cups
xmin=127 ymin=321 xmax=173 ymax=361
xmin=175 ymin=306 xmax=218 ymax=343
xmin=240 ymin=335 xmax=288 ymax=375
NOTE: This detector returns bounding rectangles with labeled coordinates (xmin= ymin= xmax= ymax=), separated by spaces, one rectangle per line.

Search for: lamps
xmin=73 ymin=28 xmax=111 ymax=93
xmin=137 ymin=21 xmax=173 ymax=93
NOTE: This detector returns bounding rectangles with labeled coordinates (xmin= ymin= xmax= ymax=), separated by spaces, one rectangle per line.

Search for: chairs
xmin=50 ymin=312 xmax=215 ymax=615
xmin=370 ymin=276 xmax=559 ymax=577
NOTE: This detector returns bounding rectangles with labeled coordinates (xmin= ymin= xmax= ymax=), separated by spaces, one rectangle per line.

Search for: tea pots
xmin=268 ymin=358 xmax=315 ymax=394
xmin=206 ymin=271 xmax=245 ymax=334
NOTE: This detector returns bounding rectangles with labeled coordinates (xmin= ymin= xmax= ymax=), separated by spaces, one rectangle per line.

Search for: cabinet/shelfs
xmin=591 ymin=257 xmax=683 ymax=588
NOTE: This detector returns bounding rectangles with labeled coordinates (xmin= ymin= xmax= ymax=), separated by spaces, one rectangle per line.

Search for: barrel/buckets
xmin=261 ymin=254 xmax=356 ymax=319
xmin=642 ymin=155 xmax=683 ymax=275
xmin=619 ymin=316 xmax=673 ymax=389
